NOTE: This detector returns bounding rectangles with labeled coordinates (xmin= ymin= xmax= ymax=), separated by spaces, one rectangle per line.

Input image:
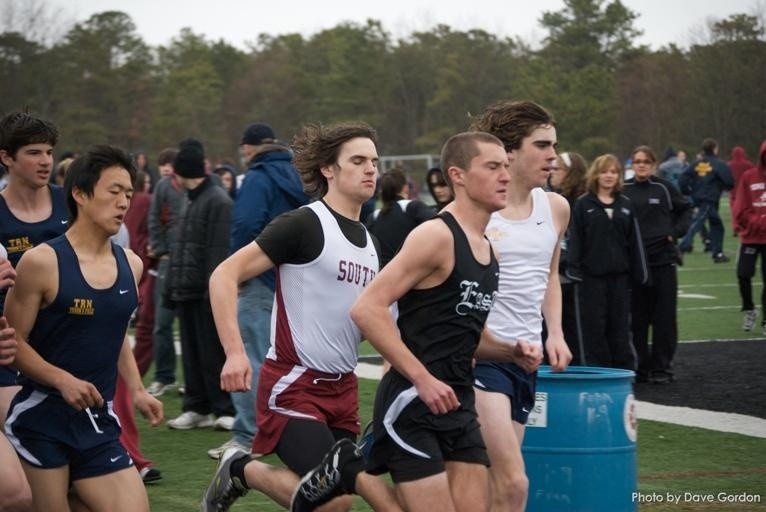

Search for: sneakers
xmin=635 ymin=371 xmax=676 ymax=384
xmin=290 ymin=437 xmax=369 ymax=512
xmin=208 ymin=437 xmax=264 ymax=458
xmin=147 ymin=379 xmax=186 ymax=396
xmin=166 ymin=410 xmax=213 ymax=429
xmin=742 ymin=308 xmax=766 ymax=334
xmin=202 ymin=446 xmax=252 ymax=512
xmin=680 ymin=242 xmax=730 ymax=263
xmin=141 ymin=468 xmax=162 ymax=483
xmin=213 ymin=415 xmax=238 ymax=431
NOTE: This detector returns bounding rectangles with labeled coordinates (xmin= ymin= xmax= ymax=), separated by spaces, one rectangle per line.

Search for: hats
xmin=173 ymin=147 xmax=206 ymax=179
xmin=239 ymin=122 xmax=276 ymax=144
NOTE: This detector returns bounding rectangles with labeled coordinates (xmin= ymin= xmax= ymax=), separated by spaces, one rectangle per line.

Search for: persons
xmin=208 ymin=120 xmax=384 ymax=512
xmin=357 ymin=163 xmax=455 ymax=370
xmin=468 ymin=96 xmax=572 ymax=512
xmin=289 ymin=130 xmax=513 ymax=512
xmin=1 ymin=105 xmax=311 ymax=512
xmin=542 ymin=134 xmax=766 ymax=389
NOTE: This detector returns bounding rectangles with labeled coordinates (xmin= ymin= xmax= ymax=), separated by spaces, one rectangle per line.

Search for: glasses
xmin=631 ymin=158 xmax=655 ymax=165
